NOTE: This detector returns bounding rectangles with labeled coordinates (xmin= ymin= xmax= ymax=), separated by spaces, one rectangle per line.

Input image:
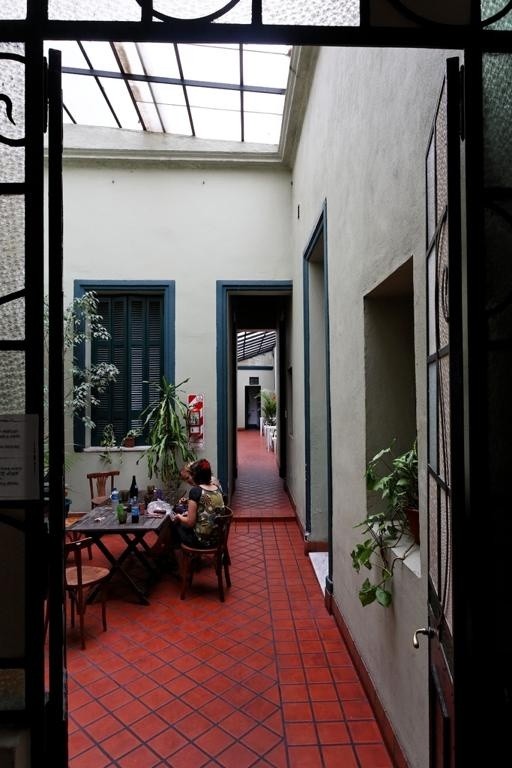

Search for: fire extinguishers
xmin=189 ymin=404 xmax=201 ymax=437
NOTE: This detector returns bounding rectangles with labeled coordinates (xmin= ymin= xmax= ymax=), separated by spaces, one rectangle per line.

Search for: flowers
xmin=99 ymin=424 xmax=116 ymax=464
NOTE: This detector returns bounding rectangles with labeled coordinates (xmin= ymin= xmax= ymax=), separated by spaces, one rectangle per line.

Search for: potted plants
xmin=349 ymin=434 xmax=420 ymax=611
xmin=120 ymin=428 xmax=144 ymax=466
xmin=44 ymin=444 xmax=89 ymax=518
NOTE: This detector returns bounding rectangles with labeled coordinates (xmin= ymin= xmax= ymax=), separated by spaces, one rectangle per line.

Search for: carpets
xmin=309 ymin=551 xmax=329 ymax=595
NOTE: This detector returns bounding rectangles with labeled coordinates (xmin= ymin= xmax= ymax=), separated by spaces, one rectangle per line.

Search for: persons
xmin=141 ymin=460 xmax=224 ymax=567
xmin=154 ymin=457 xmax=228 ymax=578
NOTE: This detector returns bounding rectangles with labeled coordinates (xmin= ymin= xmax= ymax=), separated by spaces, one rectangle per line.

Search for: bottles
xmin=111 ymin=486 xmax=119 ymax=512
xmin=128 ymin=474 xmax=139 ymax=495
xmin=131 ymin=496 xmax=139 ymax=523
xmin=116 ymin=498 xmax=127 ymax=523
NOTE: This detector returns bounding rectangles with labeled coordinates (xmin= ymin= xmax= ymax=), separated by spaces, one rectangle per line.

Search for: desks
xmin=260 ymin=417 xmax=276 ymax=453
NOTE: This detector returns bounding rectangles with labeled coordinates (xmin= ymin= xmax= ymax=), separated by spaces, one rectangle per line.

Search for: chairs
xmin=65 ymin=471 xmax=234 ymax=650
